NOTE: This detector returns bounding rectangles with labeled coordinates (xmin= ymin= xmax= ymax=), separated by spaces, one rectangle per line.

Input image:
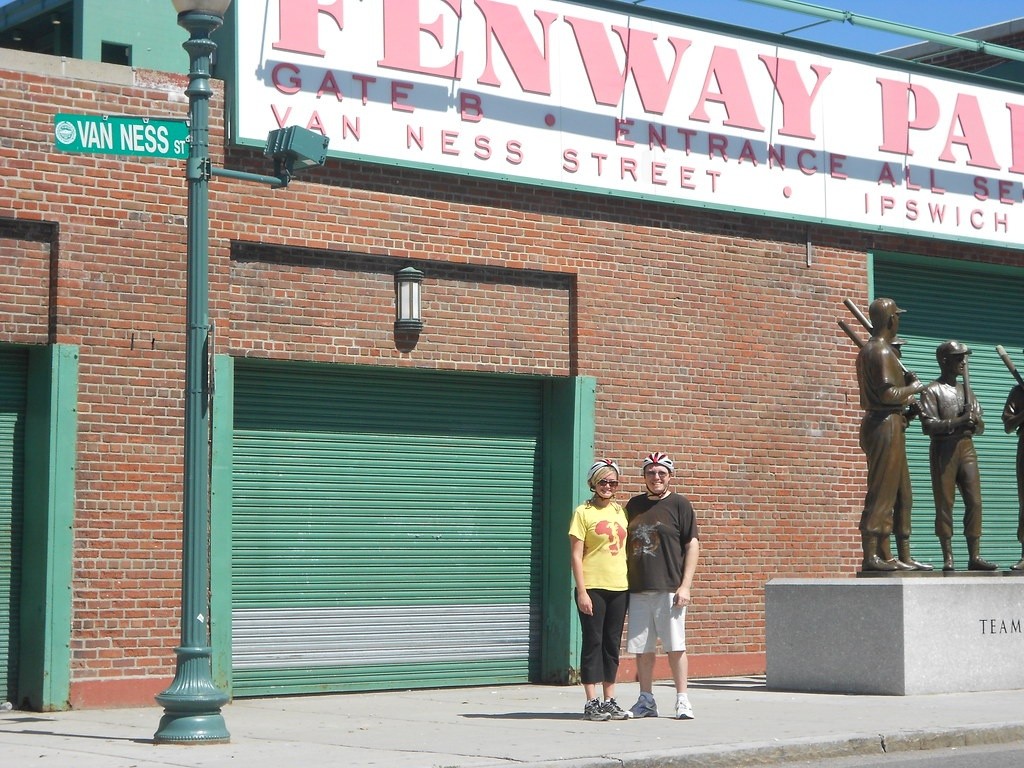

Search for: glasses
xmin=596 ymin=478 xmax=619 ymax=487
xmin=646 ymin=469 xmax=670 ymax=478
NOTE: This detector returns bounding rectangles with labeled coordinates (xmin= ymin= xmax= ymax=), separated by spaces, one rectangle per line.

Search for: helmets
xmin=587 ymin=459 xmax=619 ymax=479
xmin=643 ymin=451 xmax=675 ymax=475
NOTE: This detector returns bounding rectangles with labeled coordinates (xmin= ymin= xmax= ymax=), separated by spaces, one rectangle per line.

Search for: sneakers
xmin=601 ymin=697 xmax=629 ymax=720
xmin=629 ymin=694 xmax=659 ymax=717
xmin=583 ymin=697 xmax=613 ymax=720
xmin=675 ymin=697 xmax=694 ymax=719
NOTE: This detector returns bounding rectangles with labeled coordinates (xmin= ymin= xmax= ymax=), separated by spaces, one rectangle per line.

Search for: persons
xmin=855 ymin=297 xmax=935 ymax=571
xmin=995 ymin=344 xmax=1024 ymax=571
xmin=626 ymin=452 xmax=699 ymax=719
xmin=567 ymin=457 xmax=629 ymax=721
xmin=920 ymin=339 xmax=999 ymax=570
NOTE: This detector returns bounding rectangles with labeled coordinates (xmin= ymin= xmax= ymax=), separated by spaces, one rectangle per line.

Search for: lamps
xmin=393 ymin=267 xmax=422 ymax=331
